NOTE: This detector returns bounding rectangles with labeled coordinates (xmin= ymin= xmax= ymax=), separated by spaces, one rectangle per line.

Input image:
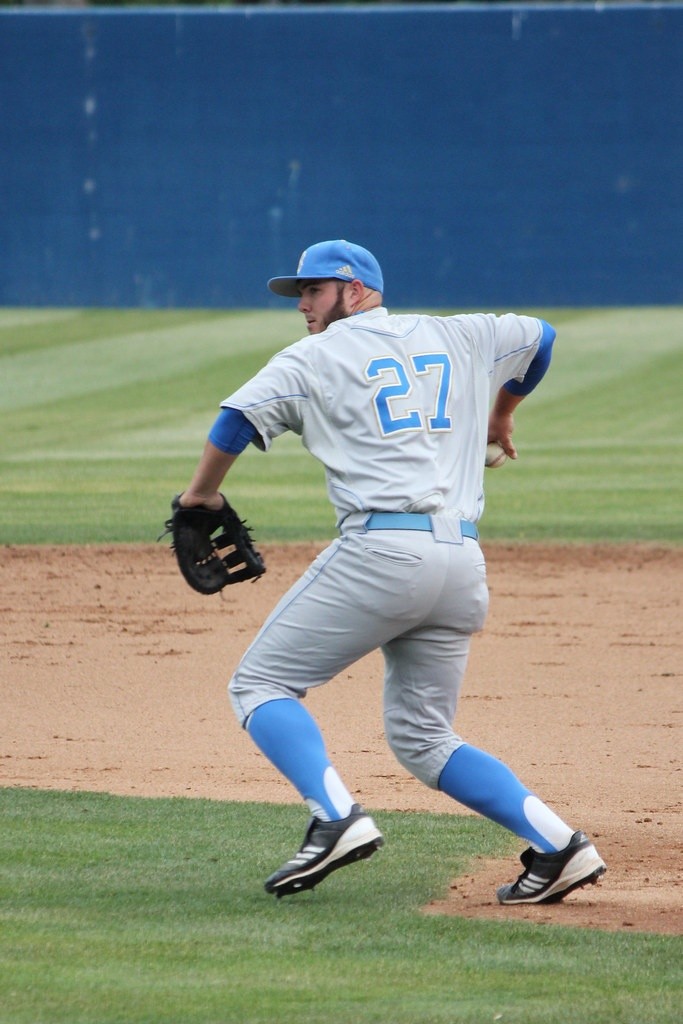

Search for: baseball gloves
xmin=156 ymin=489 xmax=268 ymax=596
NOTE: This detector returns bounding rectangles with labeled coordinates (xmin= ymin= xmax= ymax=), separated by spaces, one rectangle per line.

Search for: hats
xmin=268 ymin=240 xmax=384 ymax=296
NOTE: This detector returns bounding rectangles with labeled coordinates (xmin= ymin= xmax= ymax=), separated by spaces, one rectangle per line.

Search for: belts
xmin=367 ymin=512 xmax=477 ymax=539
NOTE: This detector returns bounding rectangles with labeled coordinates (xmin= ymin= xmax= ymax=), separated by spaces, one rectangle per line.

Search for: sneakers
xmin=497 ymin=829 xmax=608 ymax=905
xmin=262 ymin=802 xmax=385 ymax=899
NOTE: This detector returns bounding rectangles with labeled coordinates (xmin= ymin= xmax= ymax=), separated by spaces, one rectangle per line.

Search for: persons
xmin=180 ymin=239 xmax=607 ymax=906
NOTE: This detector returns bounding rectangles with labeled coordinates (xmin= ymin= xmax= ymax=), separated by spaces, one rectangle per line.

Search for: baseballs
xmin=485 ymin=442 xmax=507 ymax=469
